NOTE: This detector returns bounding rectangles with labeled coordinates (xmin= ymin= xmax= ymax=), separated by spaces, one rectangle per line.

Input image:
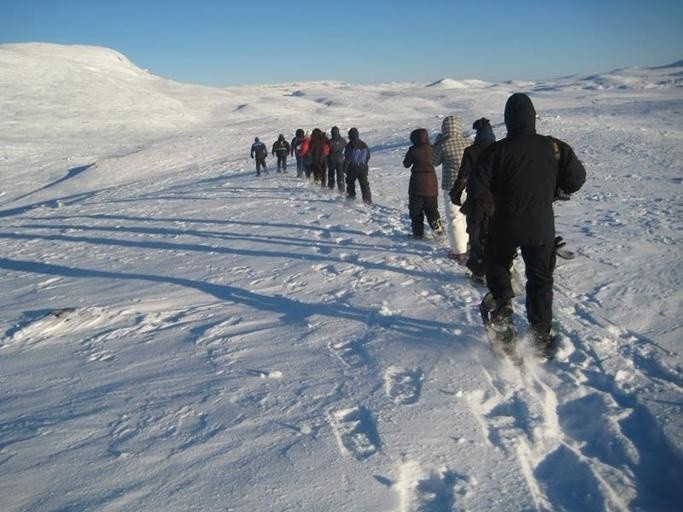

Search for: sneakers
xmin=492 ymin=299 xmax=513 ymax=322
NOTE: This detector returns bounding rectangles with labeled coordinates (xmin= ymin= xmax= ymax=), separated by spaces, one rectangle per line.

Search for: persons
xmin=289 ymin=127 xmax=371 ymax=204
xmin=402 ymin=128 xmax=445 ymax=239
xmin=448 ymin=117 xmax=500 ymax=283
xmin=250 ymin=136 xmax=269 ymax=177
xmin=271 ymin=133 xmax=290 ymax=174
xmin=477 ymin=93 xmax=586 ymax=347
xmin=430 ymin=115 xmax=475 ymax=261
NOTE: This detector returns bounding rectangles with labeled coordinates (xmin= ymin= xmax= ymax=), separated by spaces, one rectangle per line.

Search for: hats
xmin=473 ymin=118 xmax=495 ymax=142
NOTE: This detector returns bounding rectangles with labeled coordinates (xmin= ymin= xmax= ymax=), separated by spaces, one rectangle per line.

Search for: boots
xmin=432 ymin=218 xmax=445 ymax=240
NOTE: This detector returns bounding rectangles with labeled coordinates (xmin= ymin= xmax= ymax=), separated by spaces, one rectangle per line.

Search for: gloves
xmin=449 ymin=188 xmax=462 ymax=207
